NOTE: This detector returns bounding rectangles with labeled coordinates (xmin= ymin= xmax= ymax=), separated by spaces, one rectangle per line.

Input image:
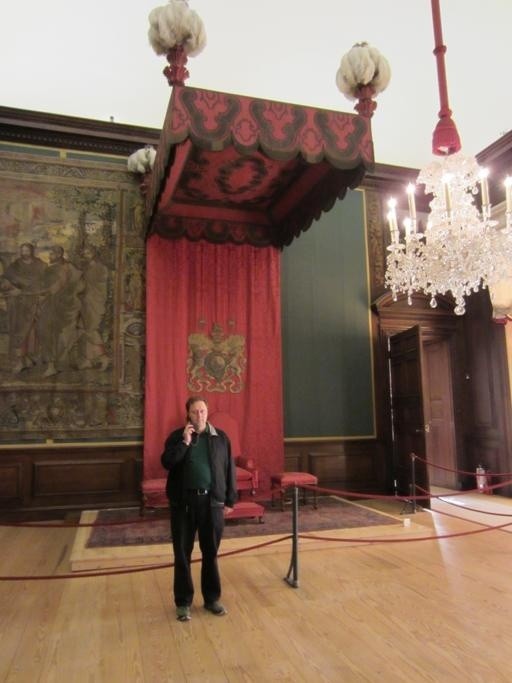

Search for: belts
xmin=183 ymin=488 xmax=212 ymax=496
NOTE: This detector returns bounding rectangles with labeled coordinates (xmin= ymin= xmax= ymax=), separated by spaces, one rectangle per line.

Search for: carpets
xmin=82 ymin=492 xmax=402 ymax=548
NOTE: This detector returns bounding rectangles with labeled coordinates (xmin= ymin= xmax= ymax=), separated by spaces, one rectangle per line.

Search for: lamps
xmin=383 ymin=1 xmax=511 ymax=314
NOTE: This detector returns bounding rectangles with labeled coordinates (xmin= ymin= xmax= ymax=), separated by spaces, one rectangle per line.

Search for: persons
xmin=2 ymin=241 xmax=110 ymax=379
xmin=160 ymin=395 xmax=238 ymax=621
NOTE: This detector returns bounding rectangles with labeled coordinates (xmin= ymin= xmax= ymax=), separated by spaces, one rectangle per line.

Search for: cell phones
xmin=186 ymin=415 xmax=198 ymax=438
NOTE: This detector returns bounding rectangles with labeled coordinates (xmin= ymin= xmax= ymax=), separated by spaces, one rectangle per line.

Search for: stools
xmin=271 ymin=471 xmax=319 ymax=512
xmin=223 ymin=500 xmax=266 ymax=524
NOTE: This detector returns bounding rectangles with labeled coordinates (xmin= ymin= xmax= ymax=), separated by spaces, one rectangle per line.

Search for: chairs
xmin=131 ymin=457 xmax=170 ymax=516
xmin=234 ymin=454 xmax=259 ymax=495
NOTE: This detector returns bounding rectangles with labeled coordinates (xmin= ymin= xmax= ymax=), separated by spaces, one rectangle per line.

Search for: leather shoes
xmin=204 ymin=601 xmax=228 ymax=616
xmin=175 ymin=606 xmax=193 ymax=621
